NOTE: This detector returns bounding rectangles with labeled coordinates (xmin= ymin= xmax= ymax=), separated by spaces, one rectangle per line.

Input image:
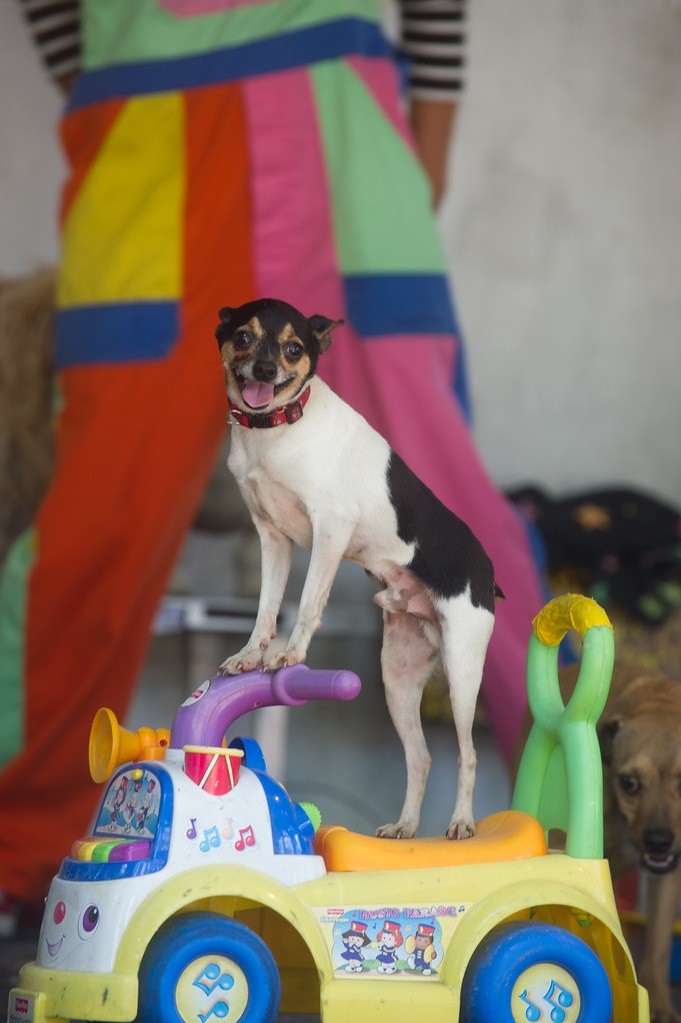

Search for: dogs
xmin=214 ymin=297 xmax=507 ymax=840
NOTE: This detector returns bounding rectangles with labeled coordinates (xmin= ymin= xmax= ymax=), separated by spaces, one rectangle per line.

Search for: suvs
xmin=4 ymin=594 xmax=651 ymax=1022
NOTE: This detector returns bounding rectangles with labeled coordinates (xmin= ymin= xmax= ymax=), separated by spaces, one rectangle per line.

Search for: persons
xmin=1 ymin=0 xmax=574 ymax=944
